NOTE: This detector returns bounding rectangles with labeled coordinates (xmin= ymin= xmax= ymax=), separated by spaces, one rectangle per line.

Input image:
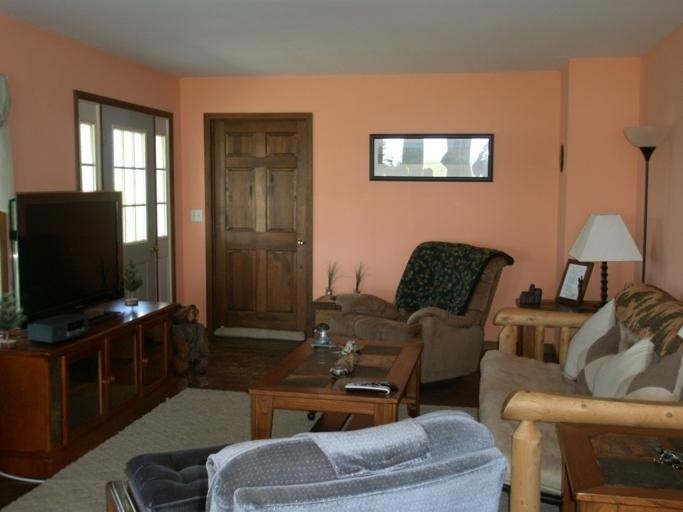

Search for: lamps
xmin=621 ymin=124 xmax=667 ymax=283
xmin=566 ymin=211 xmax=644 ymax=310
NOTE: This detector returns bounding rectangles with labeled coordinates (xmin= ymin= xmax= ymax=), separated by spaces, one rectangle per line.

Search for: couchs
xmin=476 ymin=283 xmax=682 ymax=512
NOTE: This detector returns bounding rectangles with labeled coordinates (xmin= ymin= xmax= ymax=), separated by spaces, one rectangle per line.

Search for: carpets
xmin=1 ymin=386 xmax=322 ymax=512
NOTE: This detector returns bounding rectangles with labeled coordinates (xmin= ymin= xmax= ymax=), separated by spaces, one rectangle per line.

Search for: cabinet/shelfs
xmin=0 ymin=298 xmax=187 ymax=454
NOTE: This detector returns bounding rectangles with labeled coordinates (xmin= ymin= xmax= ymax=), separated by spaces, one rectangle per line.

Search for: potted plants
xmin=1 ymin=293 xmax=27 ymax=350
xmin=123 ymin=257 xmax=143 ymax=305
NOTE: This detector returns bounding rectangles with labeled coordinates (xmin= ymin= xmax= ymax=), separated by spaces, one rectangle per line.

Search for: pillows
xmin=591 ymin=335 xmax=656 ymax=399
xmin=614 ymin=350 xmax=682 ymax=402
xmin=559 ymin=297 xmax=616 ymax=378
xmin=574 ymin=321 xmax=619 ymax=398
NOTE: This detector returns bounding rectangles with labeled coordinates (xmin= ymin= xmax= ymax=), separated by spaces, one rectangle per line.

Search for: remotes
xmin=345 ymin=381 xmax=392 ymax=396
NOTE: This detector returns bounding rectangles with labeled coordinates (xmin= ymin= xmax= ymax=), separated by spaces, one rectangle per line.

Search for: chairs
xmin=105 ymin=409 xmax=509 ymax=512
xmin=328 ymin=239 xmax=514 ymax=386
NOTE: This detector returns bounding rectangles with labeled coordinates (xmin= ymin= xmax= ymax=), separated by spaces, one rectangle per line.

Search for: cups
xmin=312 ymin=323 xmax=332 ymax=347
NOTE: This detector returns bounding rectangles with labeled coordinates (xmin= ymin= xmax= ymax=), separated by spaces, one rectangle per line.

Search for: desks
xmin=311 ymin=295 xmax=344 ymax=335
xmin=515 ymin=297 xmax=601 ymax=357
xmin=553 ymin=421 xmax=682 ymax=512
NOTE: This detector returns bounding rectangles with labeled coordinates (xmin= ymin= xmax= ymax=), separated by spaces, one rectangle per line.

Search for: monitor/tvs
xmin=6 ymin=191 xmax=123 ymax=341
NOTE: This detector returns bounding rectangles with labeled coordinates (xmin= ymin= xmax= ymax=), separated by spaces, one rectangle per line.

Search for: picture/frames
xmin=367 ymin=133 xmax=493 ymax=182
xmin=552 ymin=259 xmax=593 ymax=308
